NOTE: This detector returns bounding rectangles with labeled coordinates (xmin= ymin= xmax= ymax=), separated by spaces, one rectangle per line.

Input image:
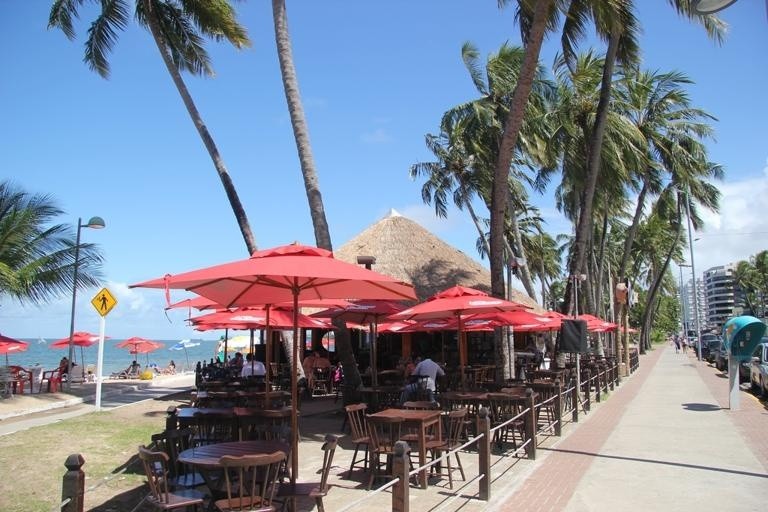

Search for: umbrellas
xmin=51 ymin=331 xmax=111 ymax=377
xmin=0 ymin=333 xmax=30 ymax=367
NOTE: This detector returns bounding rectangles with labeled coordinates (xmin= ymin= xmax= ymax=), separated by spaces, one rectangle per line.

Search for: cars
xmin=678 ymin=323 xmax=767 ymax=401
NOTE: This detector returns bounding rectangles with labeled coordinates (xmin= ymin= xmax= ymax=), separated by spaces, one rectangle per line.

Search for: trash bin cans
xmin=29 ymin=366 xmax=44 ymax=387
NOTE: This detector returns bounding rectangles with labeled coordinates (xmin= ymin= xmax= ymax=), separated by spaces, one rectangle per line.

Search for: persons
xmin=673 ymin=335 xmax=688 ymax=354
xmin=55 ymin=357 xmax=75 ymax=373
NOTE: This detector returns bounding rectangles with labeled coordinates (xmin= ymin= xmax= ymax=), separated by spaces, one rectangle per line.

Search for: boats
xmin=37 ymin=336 xmax=47 ymax=345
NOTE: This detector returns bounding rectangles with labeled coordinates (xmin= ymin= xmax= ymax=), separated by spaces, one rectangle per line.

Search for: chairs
xmin=0 ymin=364 xmax=96 ymax=394
xmin=110 ymin=366 xmax=139 ymax=379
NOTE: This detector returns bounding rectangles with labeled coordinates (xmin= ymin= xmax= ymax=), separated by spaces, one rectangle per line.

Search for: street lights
xmin=505 ymin=253 xmax=526 ymax=381
xmin=575 ymin=273 xmax=588 ymax=402
xmin=678 ymin=237 xmax=704 ymax=337
xmin=684 ymin=162 xmax=722 ymax=359
xmin=64 ymin=213 xmax=106 ymax=397
xmin=355 ymin=256 xmax=379 ymax=389
